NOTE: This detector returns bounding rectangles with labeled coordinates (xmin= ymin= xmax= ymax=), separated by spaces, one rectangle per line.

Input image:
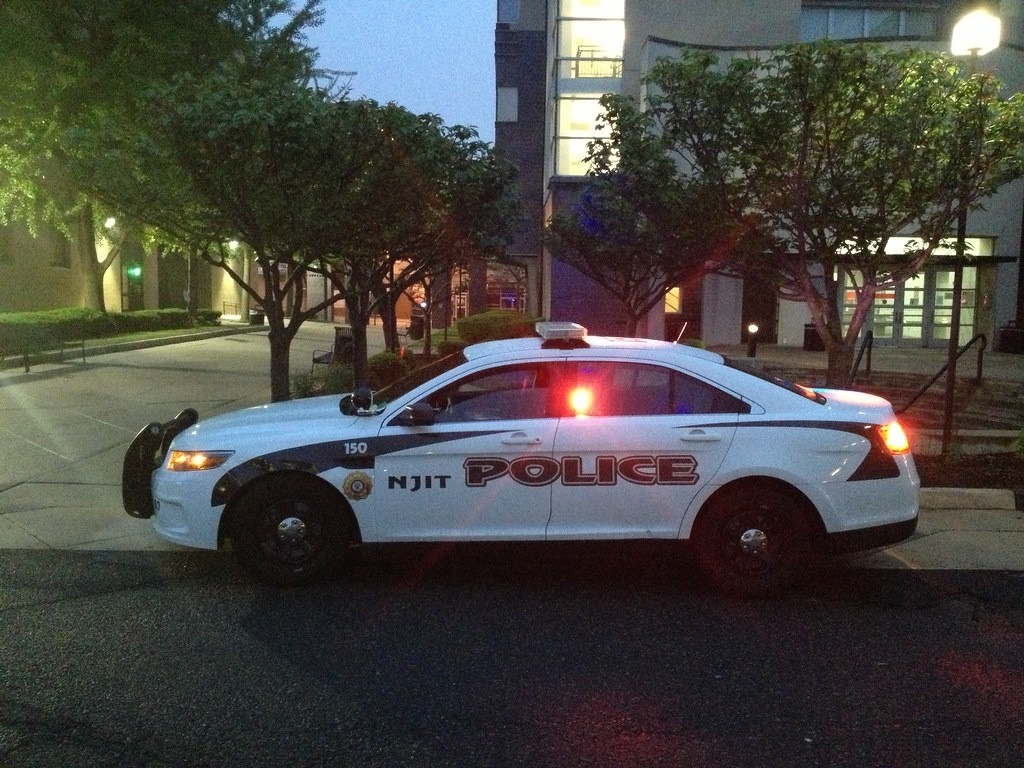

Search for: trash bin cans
xmin=999 ymin=327 xmax=1024 ymax=352
xmin=249 ymin=314 xmax=264 ymax=325
xmin=803 ymin=324 xmax=825 ymax=351
xmin=334 ymin=325 xmax=352 ymax=357
xmin=410 ymin=315 xmax=423 ymax=339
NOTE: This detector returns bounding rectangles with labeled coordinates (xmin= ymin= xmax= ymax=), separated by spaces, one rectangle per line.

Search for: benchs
xmin=0 ymin=325 xmax=91 ymax=369
xmin=308 ymin=325 xmax=355 ymax=374
xmin=396 ymin=324 xmax=415 ymax=344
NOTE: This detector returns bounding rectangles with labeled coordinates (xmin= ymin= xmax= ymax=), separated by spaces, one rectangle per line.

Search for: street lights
xmin=939 ymin=10 xmax=1003 ymax=472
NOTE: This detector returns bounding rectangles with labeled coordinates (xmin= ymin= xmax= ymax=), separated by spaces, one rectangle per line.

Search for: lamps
xmin=744 ymin=321 xmax=761 ymax=335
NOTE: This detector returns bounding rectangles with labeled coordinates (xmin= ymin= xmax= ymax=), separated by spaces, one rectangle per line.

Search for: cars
xmin=122 ymin=321 xmax=922 ymax=604
